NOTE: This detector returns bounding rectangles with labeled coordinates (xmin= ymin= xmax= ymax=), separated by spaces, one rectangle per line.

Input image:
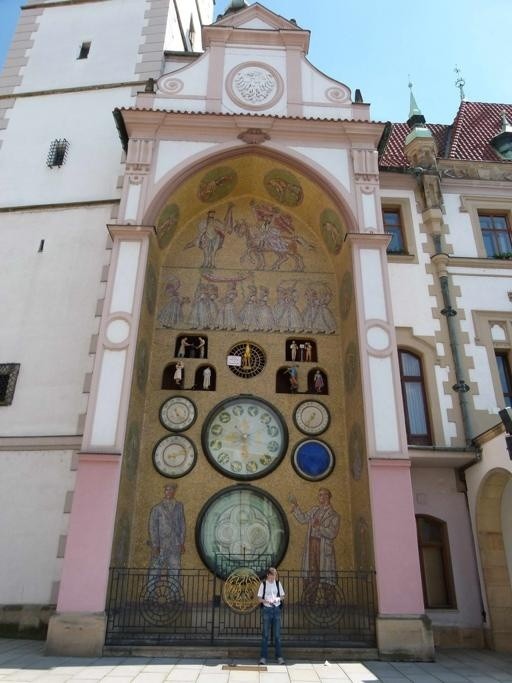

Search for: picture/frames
xmin=194 ymin=483 xmax=290 ymax=585
xmin=290 ymin=437 xmax=336 ymax=483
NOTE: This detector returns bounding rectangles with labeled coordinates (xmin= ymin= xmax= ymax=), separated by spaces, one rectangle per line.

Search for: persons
xmin=194 ymin=336 xmax=206 ymax=358
xmin=177 ymin=336 xmax=192 ymax=358
xmin=304 ymin=340 xmax=313 ymax=362
xmin=288 ymin=488 xmax=341 ymax=606
xmin=173 ymin=359 xmax=184 ymax=385
xmin=146 ymin=481 xmax=186 ymax=611
xmin=202 ymin=367 xmax=211 ymax=390
xmin=313 ymin=370 xmax=323 ymax=393
xmin=155 ymin=279 xmax=338 ymax=334
xmin=290 ymin=340 xmax=298 ymax=362
xmin=257 ymin=567 xmax=285 ymax=664
xmin=284 ymin=366 xmax=299 ymax=391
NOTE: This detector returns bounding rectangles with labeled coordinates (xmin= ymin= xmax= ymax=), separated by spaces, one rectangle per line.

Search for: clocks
xmin=157 ymin=394 xmax=199 ymax=434
xmin=200 ymin=393 xmax=289 ymax=482
xmin=292 ymin=398 xmax=332 ymax=436
xmin=150 ymin=433 xmax=199 ymax=479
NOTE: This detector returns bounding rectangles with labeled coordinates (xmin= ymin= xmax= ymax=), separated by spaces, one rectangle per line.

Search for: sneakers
xmin=277 ymin=657 xmax=284 ymax=664
xmin=260 ymin=658 xmax=266 ymax=664
